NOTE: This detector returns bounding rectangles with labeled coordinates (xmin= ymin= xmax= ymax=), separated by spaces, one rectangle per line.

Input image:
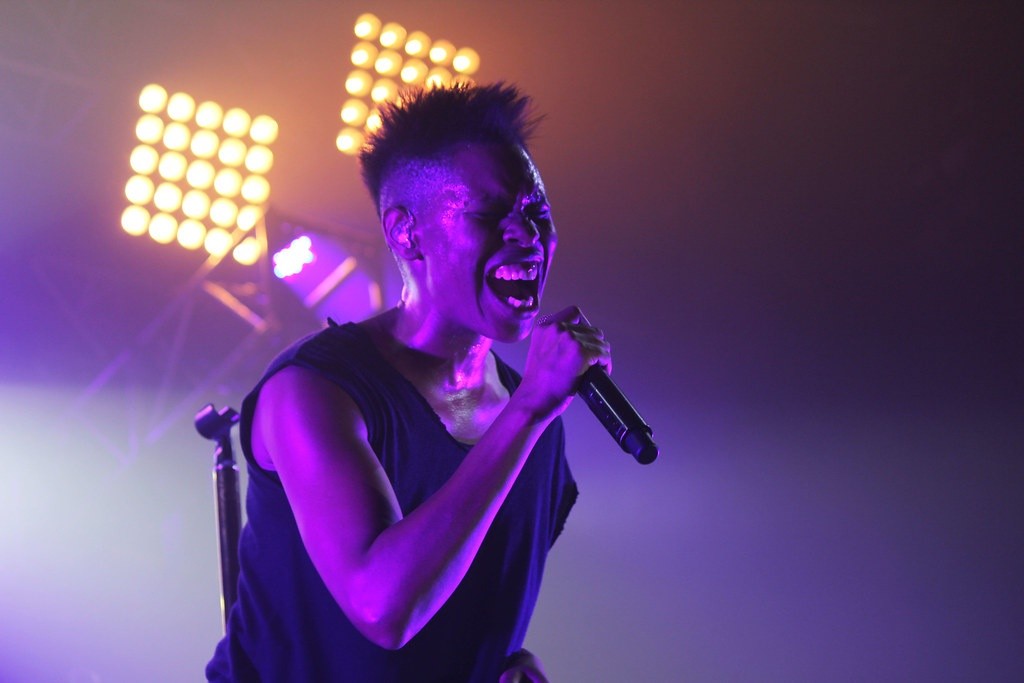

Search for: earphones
xmin=390 ymin=221 xmax=414 ymax=249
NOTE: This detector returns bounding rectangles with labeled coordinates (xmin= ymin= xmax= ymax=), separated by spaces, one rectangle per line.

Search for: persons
xmin=206 ymin=79 xmax=613 ymax=683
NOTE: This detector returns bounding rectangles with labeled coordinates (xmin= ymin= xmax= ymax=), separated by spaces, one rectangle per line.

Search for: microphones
xmin=537 ymin=313 xmax=660 ymax=464
